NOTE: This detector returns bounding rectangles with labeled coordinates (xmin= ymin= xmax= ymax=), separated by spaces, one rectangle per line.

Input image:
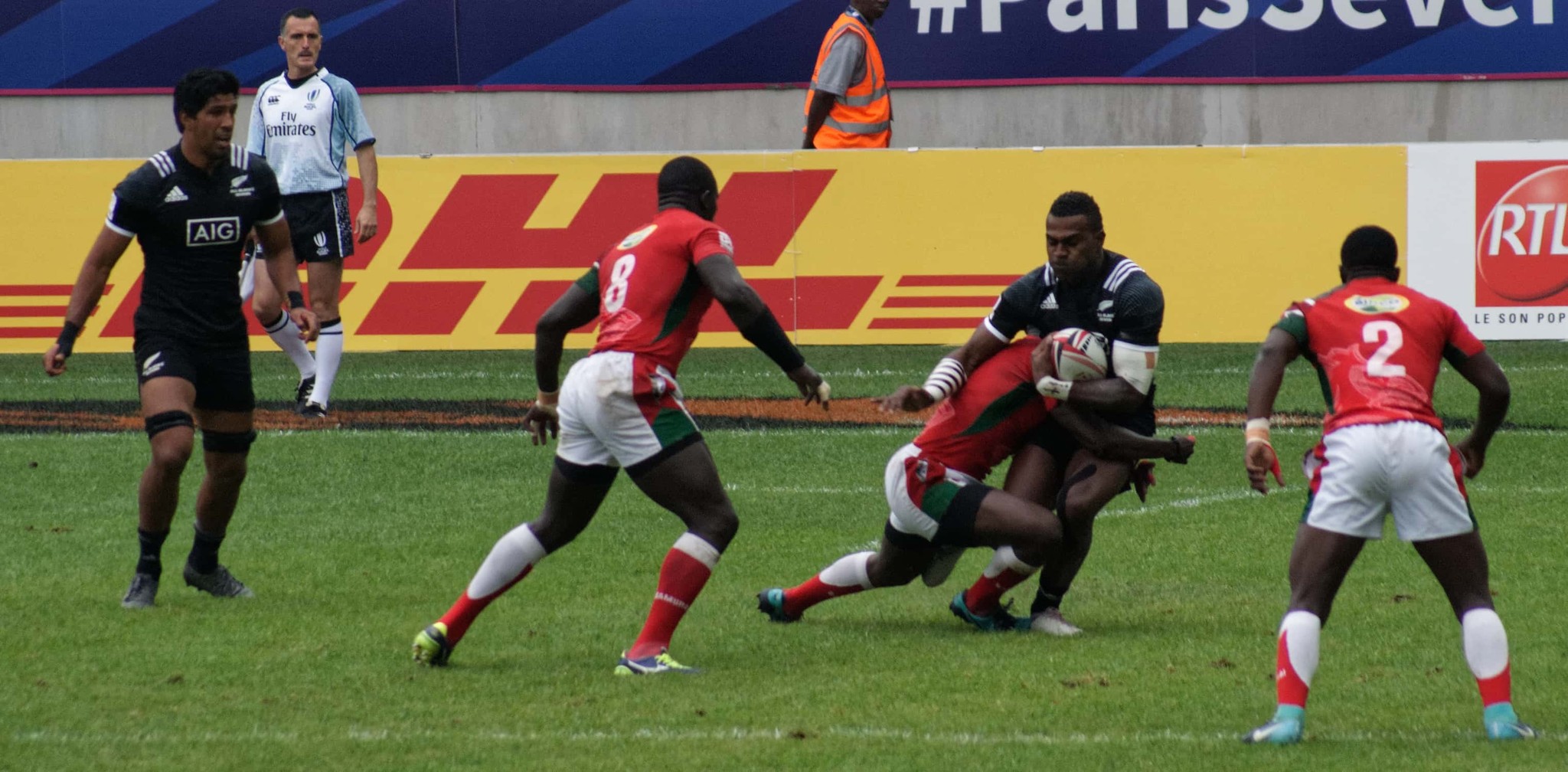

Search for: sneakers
xmin=294 ymin=374 xmax=314 ymax=414
xmin=296 ymin=401 xmax=326 ymax=419
xmin=183 ymin=558 xmax=256 ymax=600
xmin=1239 ymin=717 xmax=1302 ymax=744
xmin=757 ymin=589 xmax=807 ymax=622
xmin=948 ymin=588 xmax=1032 ymax=632
xmin=1029 ymin=608 xmax=1083 ymax=637
xmin=613 ymin=648 xmax=708 ymax=676
xmin=410 ymin=622 xmax=453 ymax=667
xmin=1485 ymin=722 xmax=1540 ymax=740
xmin=120 ymin=573 xmax=160 ymax=610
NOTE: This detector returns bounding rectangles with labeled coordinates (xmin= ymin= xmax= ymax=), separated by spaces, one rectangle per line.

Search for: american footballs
xmin=1050 ymin=326 xmax=1108 ymax=381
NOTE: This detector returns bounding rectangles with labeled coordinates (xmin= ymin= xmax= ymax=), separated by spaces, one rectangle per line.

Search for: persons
xmin=1236 ymin=223 xmax=1541 ymax=748
xmin=238 ymin=7 xmax=379 ymax=417
xmin=796 ymin=0 xmax=899 ymax=149
xmin=756 ymin=191 xmax=1202 ymax=638
xmin=410 ymin=154 xmax=834 ymax=679
xmin=43 ymin=68 xmax=321 ymax=615
